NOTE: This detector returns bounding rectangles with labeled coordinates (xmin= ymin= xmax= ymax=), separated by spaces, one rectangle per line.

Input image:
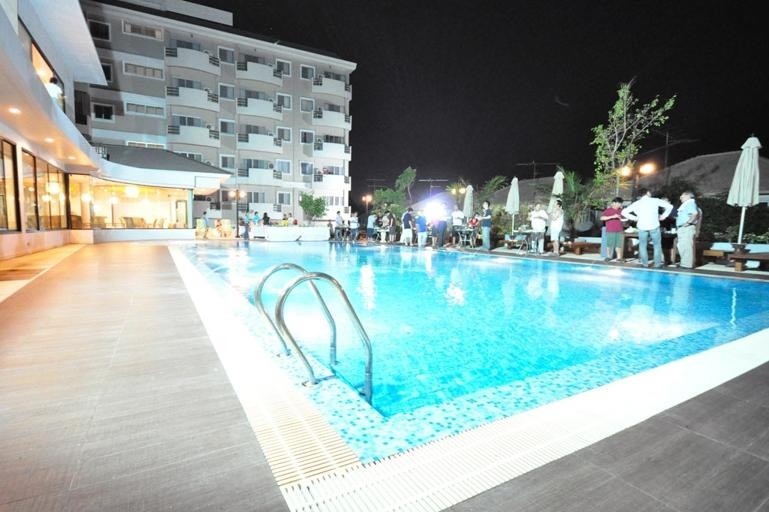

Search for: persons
xmin=200 ymin=208 xmax=448 ymax=249
xmin=467 ymin=212 xmax=481 ymax=249
xmin=448 ymin=203 xmax=466 ymax=247
xmin=529 ymin=203 xmax=549 ymax=255
xmin=599 ymin=186 xmax=703 ymax=270
xmin=476 ymin=200 xmax=492 ymax=252
xmin=548 ymin=199 xmax=565 ymax=257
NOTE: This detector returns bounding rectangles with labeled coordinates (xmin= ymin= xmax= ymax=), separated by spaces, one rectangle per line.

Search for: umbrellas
xmin=504 ymin=174 xmax=520 ymax=238
xmin=462 ymin=183 xmax=474 ymax=228
xmin=727 ymin=133 xmax=763 ymax=243
xmin=547 ymin=169 xmax=565 ymax=214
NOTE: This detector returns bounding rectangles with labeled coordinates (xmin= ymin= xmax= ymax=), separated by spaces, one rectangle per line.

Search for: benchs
xmin=330 ymin=228 xmax=769 ymax=271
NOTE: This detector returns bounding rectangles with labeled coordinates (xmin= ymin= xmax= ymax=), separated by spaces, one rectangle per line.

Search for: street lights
xmin=228 ymin=188 xmax=248 ymax=238
xmin=619 ymin=161 xmax=658 ymax=227
xmin=451 ymin=179 xmax=466 ymax=212
xmin=361 ymin=193 xmax=372 ymax=232
xmin=228 ymin=241 xmax=247 ymax=257
